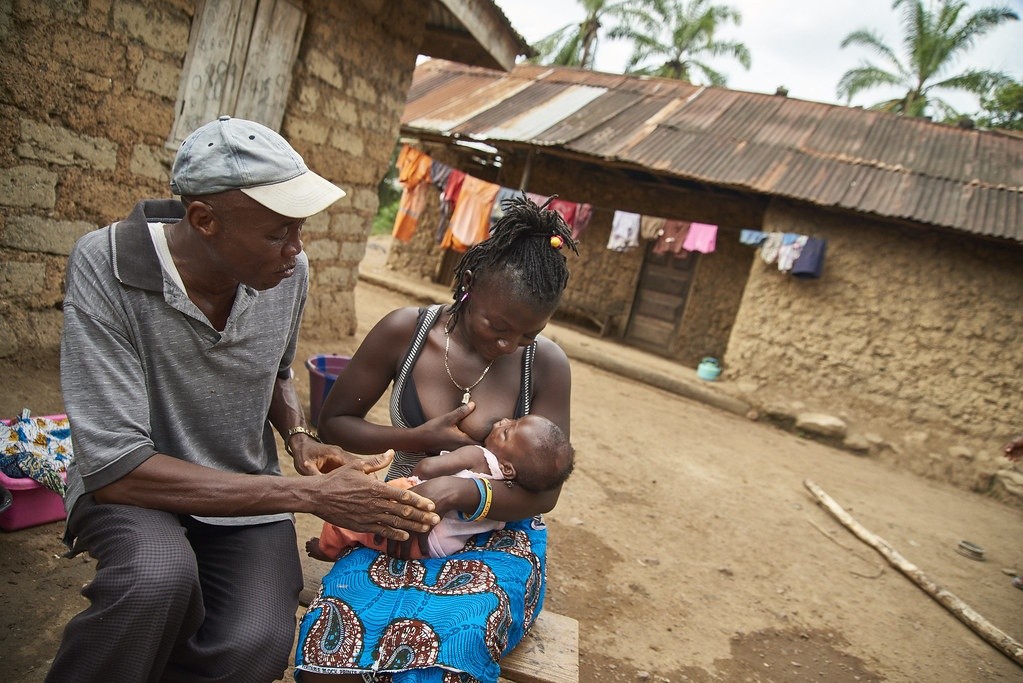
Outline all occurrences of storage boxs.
[0,412,67,532]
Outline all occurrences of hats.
[169,116,347,218]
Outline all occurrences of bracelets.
[458,478,486,521]
[285,428,322,457]
[464,478,492,522]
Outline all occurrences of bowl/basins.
[697,362,722,381]
[957,540,986,557]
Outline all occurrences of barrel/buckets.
[305,355,352,426]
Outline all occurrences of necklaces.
[444,313,495,403]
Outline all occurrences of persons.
[46,115,440,683]
[292,197,578,683]
[305,415,575,562]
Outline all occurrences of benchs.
[298,541,579,683]
